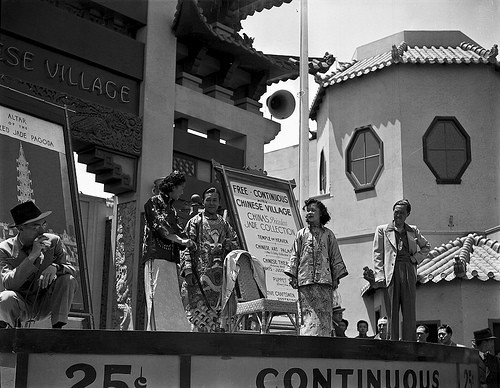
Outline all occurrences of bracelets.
[40,275,43,280]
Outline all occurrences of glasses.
[33,223,47,230]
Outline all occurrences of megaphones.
[266,90,297,119]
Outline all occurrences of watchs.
[52,263,59,271]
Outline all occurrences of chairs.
[222,250,300,336]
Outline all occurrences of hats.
[333,305,345,312]
[7,200,53,229]
[471,327,496,342]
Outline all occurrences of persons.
[0,202,77,328]
[373,200,430,340]
[332,305,348,337]
[416,326,429,343]
[437,325,455,345]
[143,172,192,332]
[373,318,387,339]
[355,320,372,338]
[473,328,500,388]
[283,198,348,337]
[182,187,240,333]
